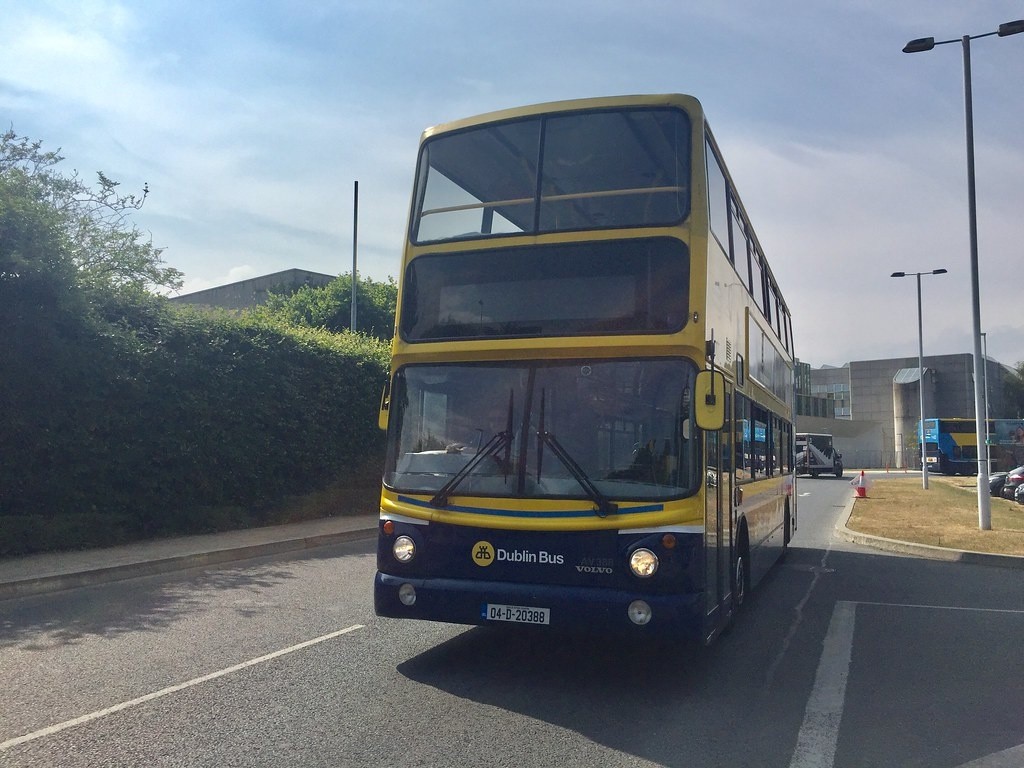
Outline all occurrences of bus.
[373,94,799,659]
[918,418,1024,477]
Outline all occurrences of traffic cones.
[853,470,870,498]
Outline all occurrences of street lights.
[900,18,1023,533]
[890,269,949,491]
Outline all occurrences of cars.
[988,465,1024,505]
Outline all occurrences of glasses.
[487,417,507,424]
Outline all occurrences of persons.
[445,409,518,461]
[1009,427,1024,445]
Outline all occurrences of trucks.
[796,432,844,479]
